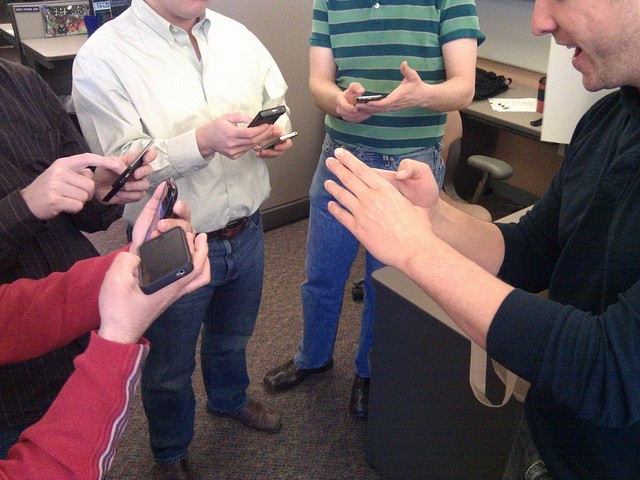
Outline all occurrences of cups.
[536,77,547,112]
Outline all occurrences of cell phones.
[143,177,178,242]
[247,105,286,125]
[136,226,195,296]
[102,140,160,202]
[256,131,299,154]
[357,95,383,103]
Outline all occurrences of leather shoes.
[350,377,369,418]
[264,357,333,389]
[206,397,282,434]
[155,456,194,480]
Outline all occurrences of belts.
[196,217,249,241]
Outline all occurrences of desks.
[0,0,89,96]
[0,22,16,42]
[453,56,569,200]
[366,205,549,478]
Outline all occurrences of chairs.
[352,139,513,301]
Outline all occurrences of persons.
[265,1,485,422]
[0,178,210,479]
[72,0,292,480]
[325,0,640,478]
[0,57,156,451]
